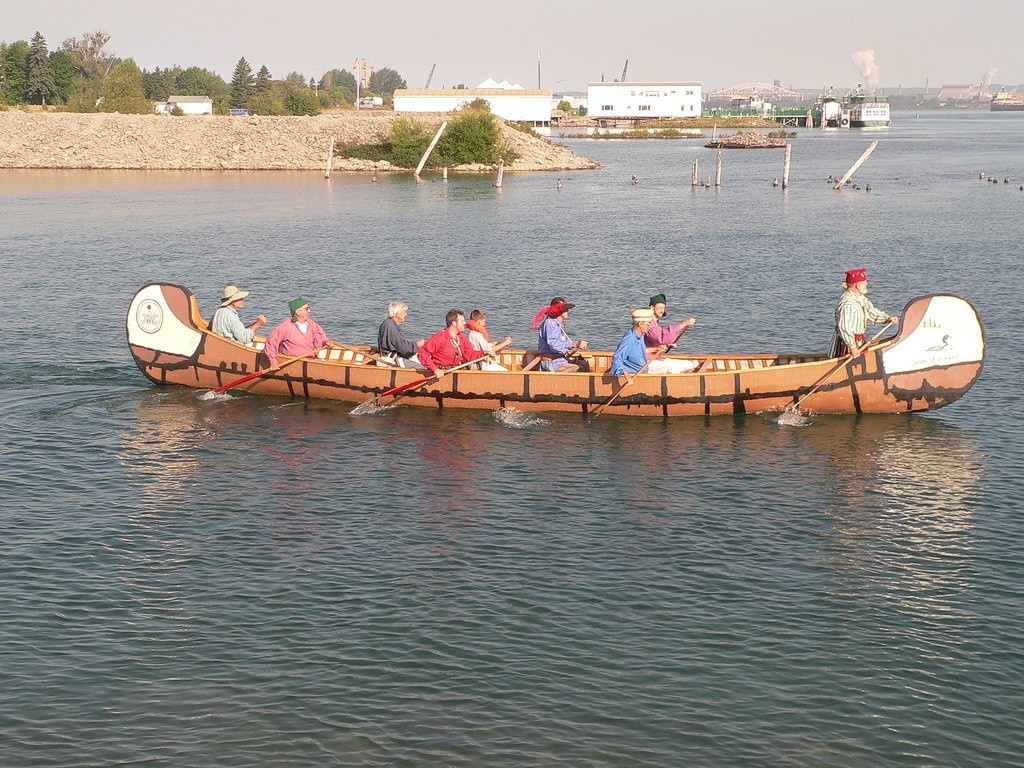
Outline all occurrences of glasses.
[302,306,310,310]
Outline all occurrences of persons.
[611,306,666,386]
[376,301,428,369]
[417,308,497,379]
[538,297,590,372]
[265,297,334,371]
[462,310,512,371]
[212,286,267,345]
[827,268,898,359]
[643,294,696,374]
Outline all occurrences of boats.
[809,94,841,127]
[989,89,1024,111]
[840,95,892,127]
[127,280,988,416]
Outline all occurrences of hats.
[844,269,867,283]
[629,307,654,322]
[219,286,249,306]
[545,297,575,317]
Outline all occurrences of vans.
[391,99,394,108]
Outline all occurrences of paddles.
[791,322,893,409]
[381,353,491,397]
[245,321,258,329]
[588,349,663,424]
[214,344,328,392]
[664,324,692,354]
[328,338,400,368]
[567,343,588,358]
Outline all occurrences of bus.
[226,108,250,116]
